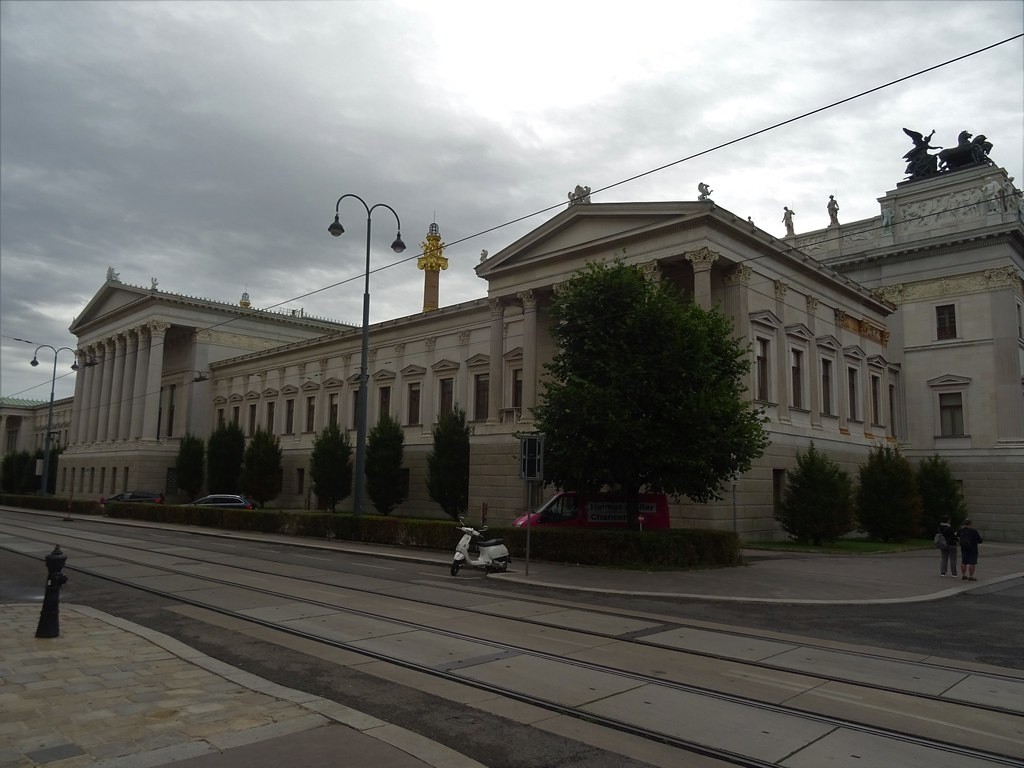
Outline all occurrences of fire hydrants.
[33,541,68,639]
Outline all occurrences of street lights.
[29,344,79,509]
[326,192,408,539]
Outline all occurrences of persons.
[747,216,754,225]
[826,195,840,227]
[782,207,795,236]
[937,515,960,577]
[956,518,982,581]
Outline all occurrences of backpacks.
[959,536,978,556]
[933,525,949,551]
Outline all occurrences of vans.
[513,489,672,534]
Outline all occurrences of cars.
[180,493,259,511]
[103,490,164,504]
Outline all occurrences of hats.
[942,515,952,521]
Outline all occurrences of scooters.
[449,516,512,577]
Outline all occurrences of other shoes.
[969,577,977,581]
[940,572,946,576]
[953,574,957,578]
[962,575,968,580]
